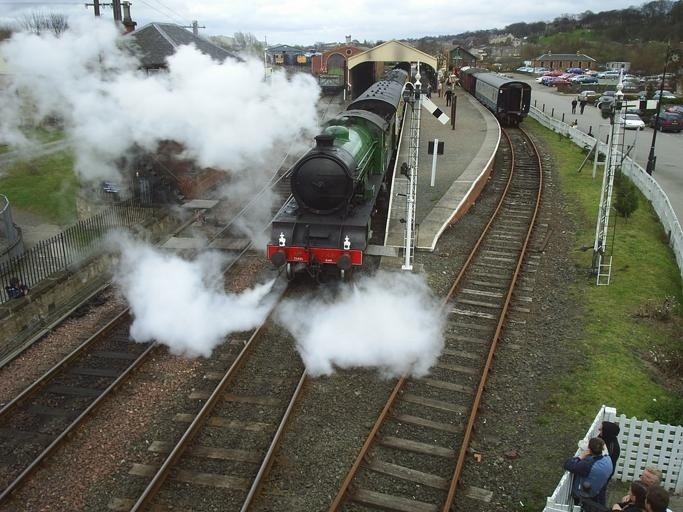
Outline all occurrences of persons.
[578,96,586,115]
[5,278,30,299]
[425,80,457,106]
[563,421,673,512]
[571,97,577,114]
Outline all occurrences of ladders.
[597,99,628,287]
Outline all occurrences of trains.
[458,63,531,126]
[266,62,412,281]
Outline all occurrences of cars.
[517,66,683,132]
